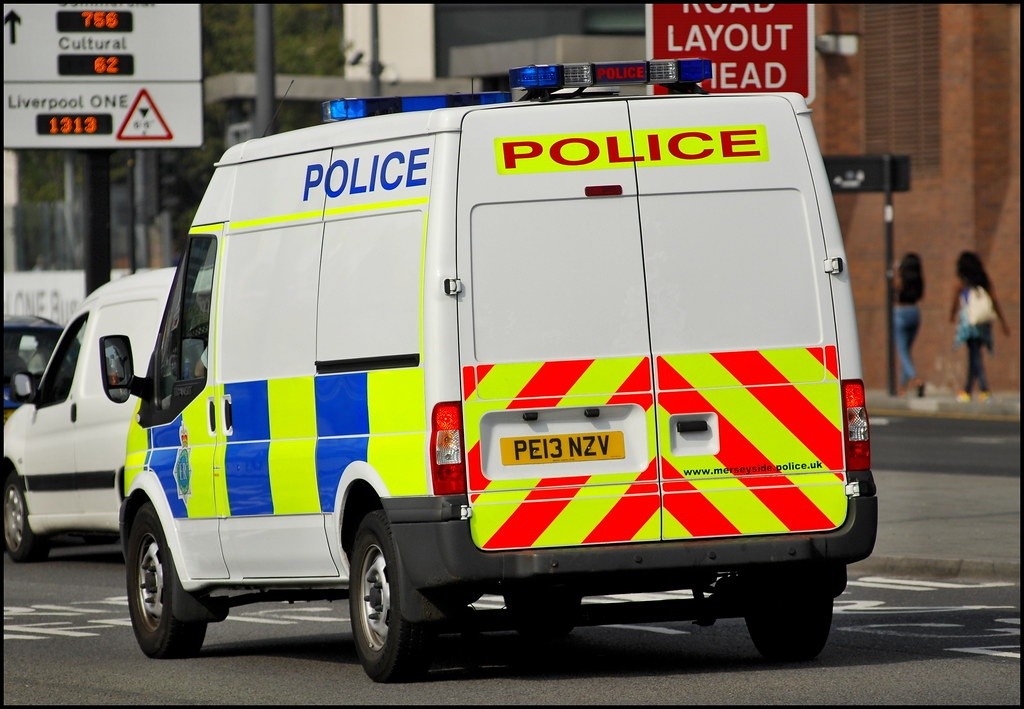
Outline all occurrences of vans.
[99,57,879,683]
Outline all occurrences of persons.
[195,348,208,376]
[950,249,1009,403]
[893,253,925,398]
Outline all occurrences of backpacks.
[959,287,997,326]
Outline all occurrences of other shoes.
[955,389,971,402]
[916,377,924,396]
[897,386,905,396]
[978,392,993,403]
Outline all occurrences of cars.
[3,314,81,425]
[3,266,176,562]
